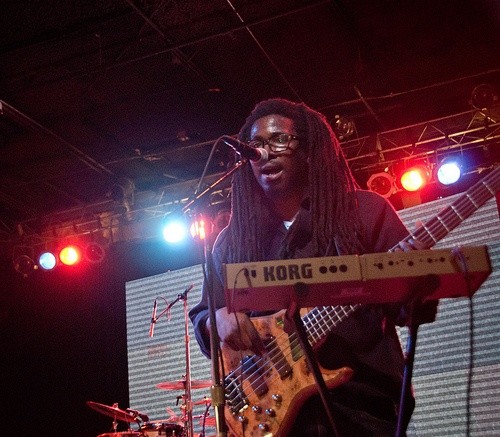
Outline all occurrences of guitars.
[215,168,500,437]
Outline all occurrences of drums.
[96,420,188,437]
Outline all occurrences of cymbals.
[87,400,142,424]
[156,380,212,390]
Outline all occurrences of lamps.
[13,228,106,278]
[366,151,469,198]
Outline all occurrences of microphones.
[127,409,149,422]
[149,300,157,338]
[223,135,268,166]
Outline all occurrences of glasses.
[248,133,310,153]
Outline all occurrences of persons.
[189,98,440,437]
